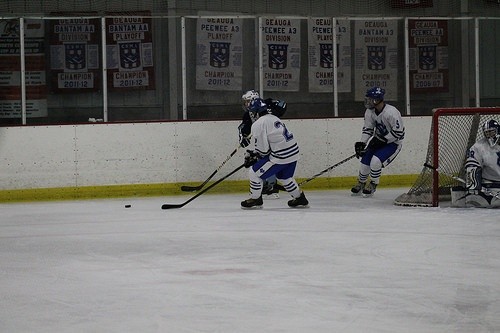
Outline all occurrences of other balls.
[125,205,131,208]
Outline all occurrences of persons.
[451,119,500,209]
[240,98,310,208]
[351,86,406,198]
[238,90,287,200]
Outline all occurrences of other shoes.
[249,179,279,199]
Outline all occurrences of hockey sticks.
[423,163,500,199]
[272,154,357,192]
[181,146,241,192]
[161,163,245,209]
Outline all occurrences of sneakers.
[361,181,378,197]
[240,193,264,210]
[350,180,366,196]
[287,191,310,209]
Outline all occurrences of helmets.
[249,98,267,120]
[241,90,259,110]
[364,87,385,110]
[482,120,500,148]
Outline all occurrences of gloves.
[367,134,387,150]
[239,134,251,148]
[244,150,258,168]
[355,142,366,159]
